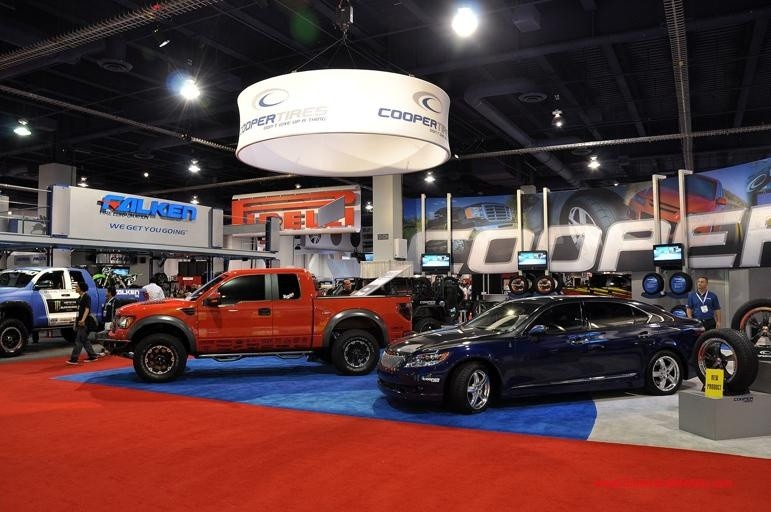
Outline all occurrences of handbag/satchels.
[85,314,98,332]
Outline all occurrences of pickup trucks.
[98,267,415,382]
[1,266,148,358]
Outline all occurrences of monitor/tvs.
[364,253,374,260]
[653,243,683,263]
[517,250,547,269]
[110,267,129,276]
[420,253,450,269]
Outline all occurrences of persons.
[685,276,721,330]
[95,285,118,358]
[65,281,99,365]
[141,278,197,302]
[326,278,358,297]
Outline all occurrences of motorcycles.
[93,265,146,289]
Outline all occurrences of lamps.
[230,2,452,180]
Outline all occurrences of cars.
[626,174,728,239]
[376,294,707,414]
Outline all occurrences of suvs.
[426,203,517,262]
[318,278,356,297]
[328,273,462,334]
